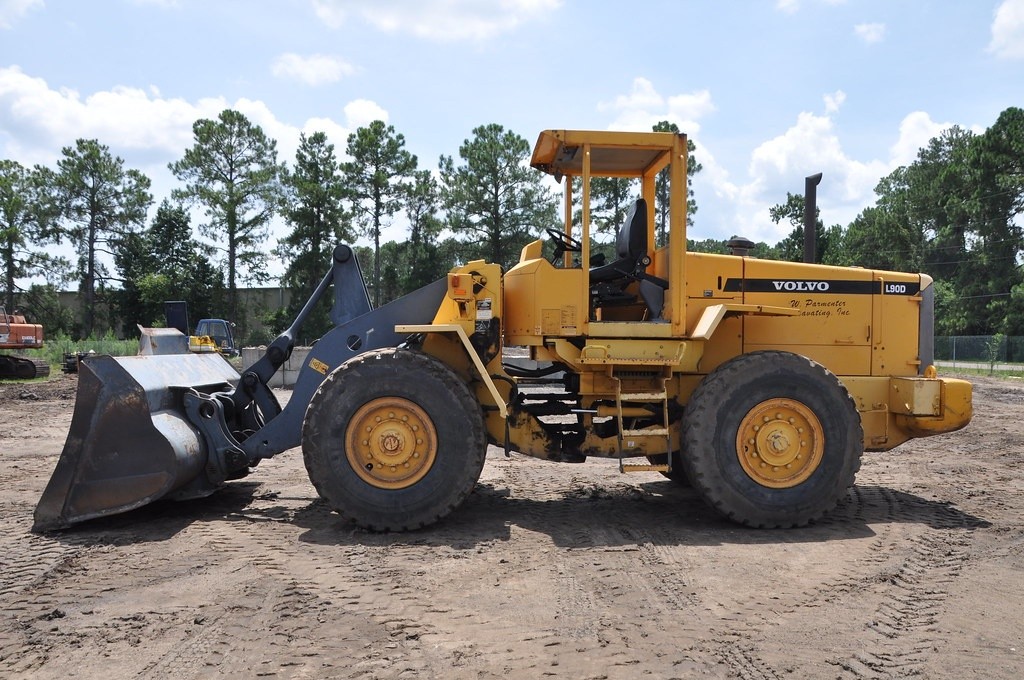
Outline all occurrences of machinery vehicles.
[25,129,979,528]
[0,306,50,379]
[185,318,235,358]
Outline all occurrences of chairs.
[588,198,649,290]
